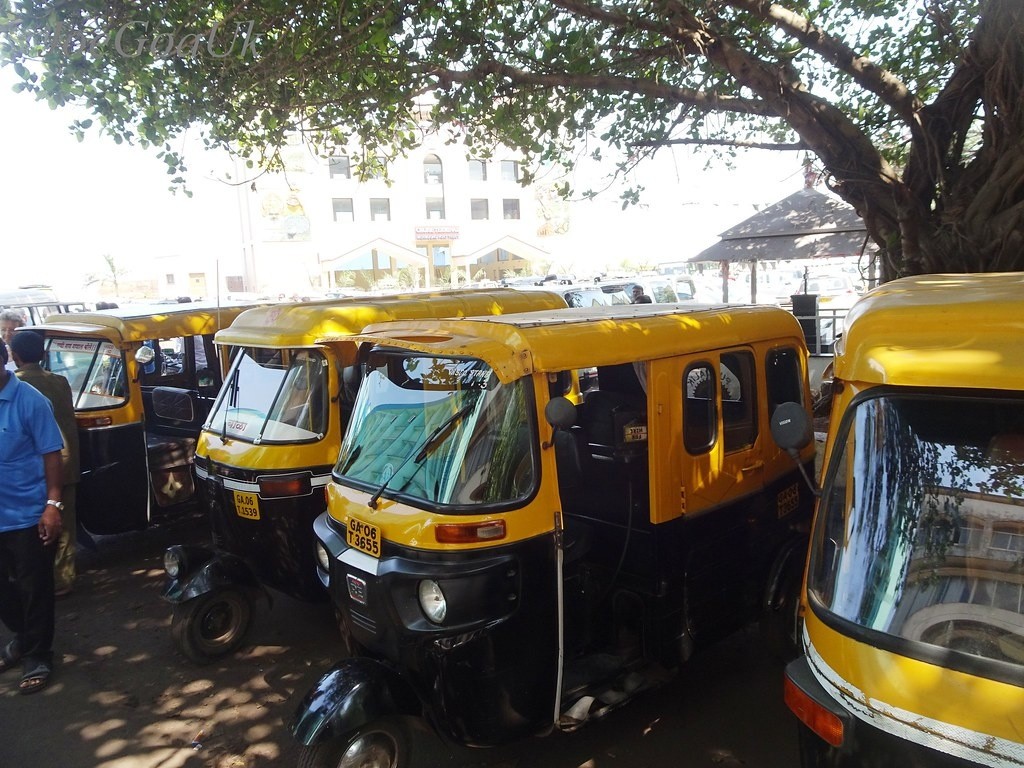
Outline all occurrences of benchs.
[139,384,200,472]
[579,446,652,578]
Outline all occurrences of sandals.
[19,659,52,696]
[0,638,22,674]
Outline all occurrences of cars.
[520,275,714,310]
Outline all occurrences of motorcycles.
[284,303,817,768]
[0,289,570,768]
[771,270,1024,768]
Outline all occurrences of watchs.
[46,499,65,511]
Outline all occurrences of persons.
[0,338,65,693]
[9,331,89,595]
[593,276,600,285]
[95,301,120,371]
[631,284,652,303]
[177,297,207,374]
[534,279,573,286]
[0,310,27,372]
[264,293,311,302]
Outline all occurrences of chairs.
[581,389,639,446]
